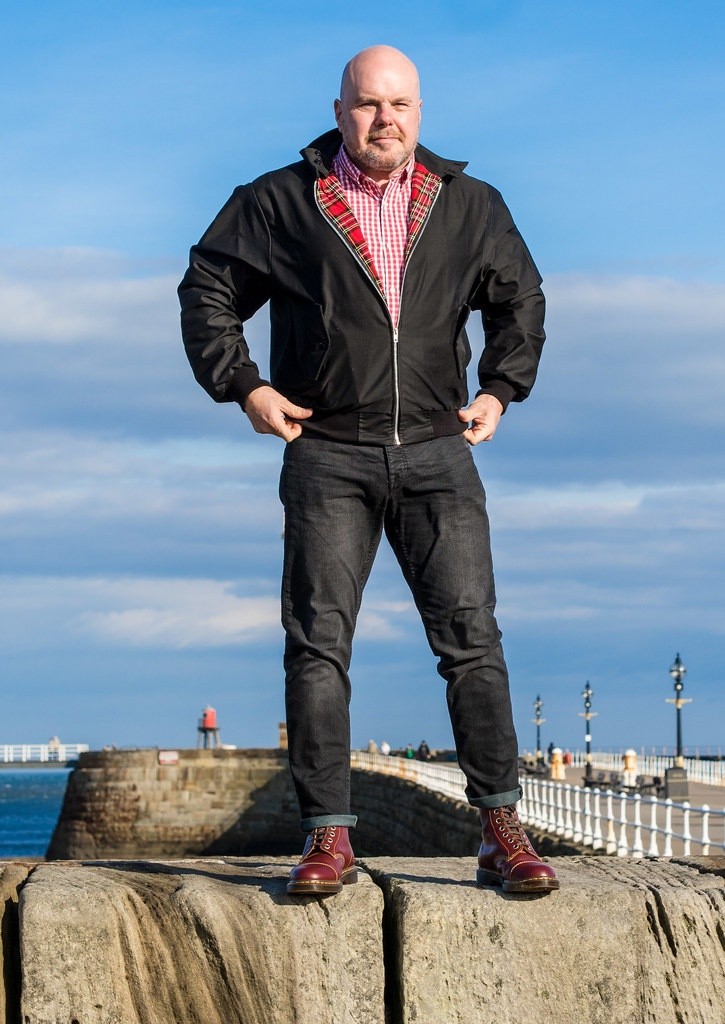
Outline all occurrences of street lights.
[666,653,693,770]
[578,681,598,790]
[532,694,546,774]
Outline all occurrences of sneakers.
[476,803,560,893]
[285,826,358,892]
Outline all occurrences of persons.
[177,46,559,899]
